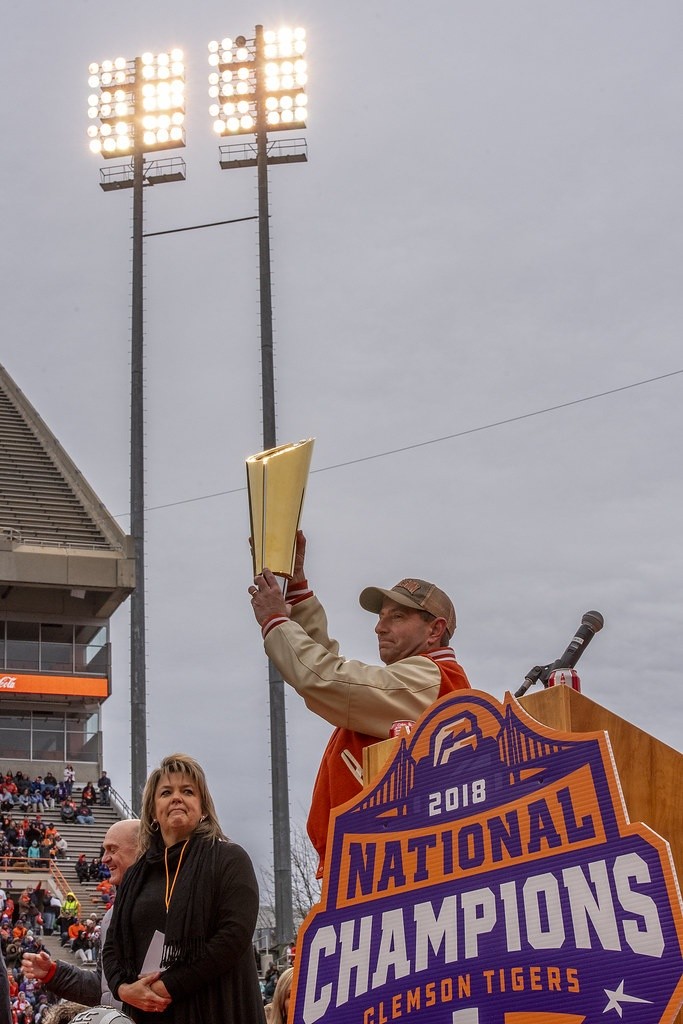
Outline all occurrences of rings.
[21,967,25,973]
[154,1007,158,1012]
[251,589,257,594]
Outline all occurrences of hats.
[0,765,293,1024]
[359,578,455,637]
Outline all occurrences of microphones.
[548,610,604,680]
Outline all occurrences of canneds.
[388,720,416,740]
[547,668,581,695]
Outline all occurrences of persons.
[0,763,116,1024]
[102,754,267,1024]
[20,819,149,1014]
[248,530,471,882]
[264,942,297,1024]
